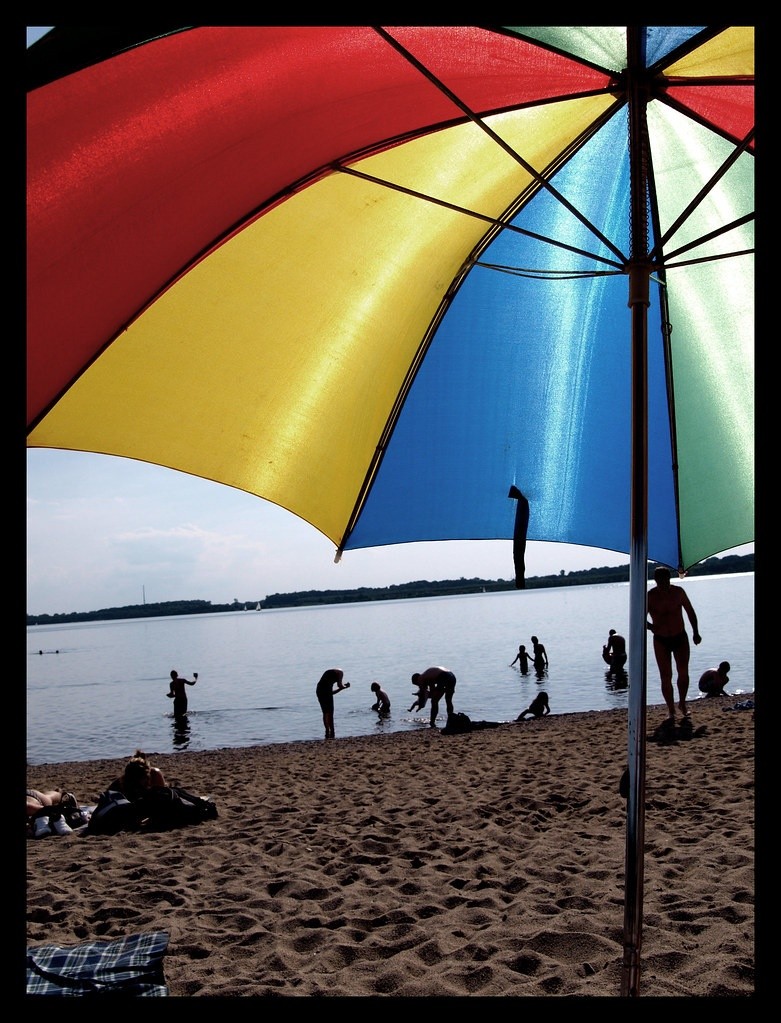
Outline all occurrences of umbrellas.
[28,25,753,995]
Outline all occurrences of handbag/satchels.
[85,787,219,834]
[441,712,472,735]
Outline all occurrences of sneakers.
[34,816,51,836]
[54,814,73,835]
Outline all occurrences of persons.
[316,669,350,738]
[531,635,548,665]
[407,666,456,727]
[166,669,199,716]
[647,566,701,718]
[698,662,731,697]
[510,645,534,674]
[515,691,550,721]
[602,629,627,673]
[89,752,165,831]
[27,788,78,835]
[370,683,391,712]
